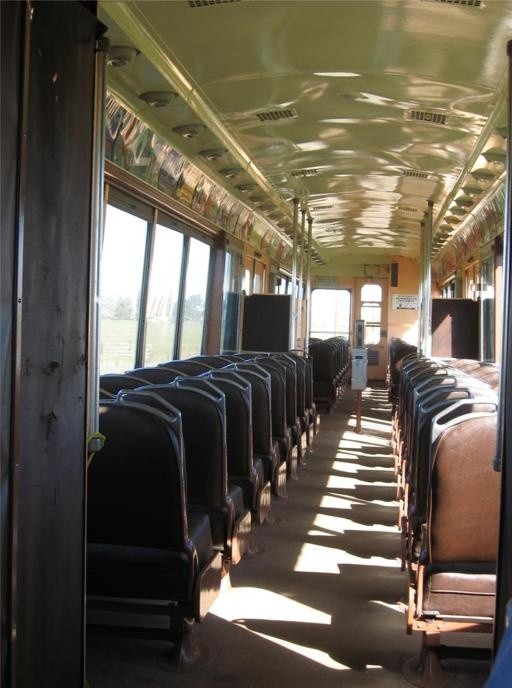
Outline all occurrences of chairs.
[384,338,509,688]
[93,334,350,675]
